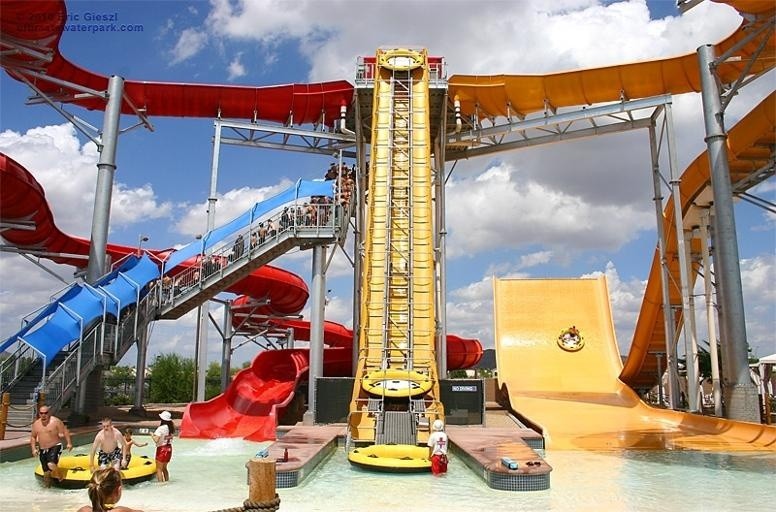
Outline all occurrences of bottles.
[283,448,288,462]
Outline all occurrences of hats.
[158,411,172,421]
[434,420,444,431]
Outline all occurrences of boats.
[33,452,157,491]
[379,48,423,72]
[361,370,434,402]
[557,329,585,352]
[348,441,433,472]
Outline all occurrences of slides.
[180,350,300,443]
[352,48,446,469]
[493,278,775,457]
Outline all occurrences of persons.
[150,410,172,484]
[73,467,146,512]
[119,159,357,319]
[122,428,148,469]
[427,420,448,476]
[30,405,72,487]
[89,416,126,472]
[561,325,581,348]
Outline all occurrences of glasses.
[39,411,48,414]
